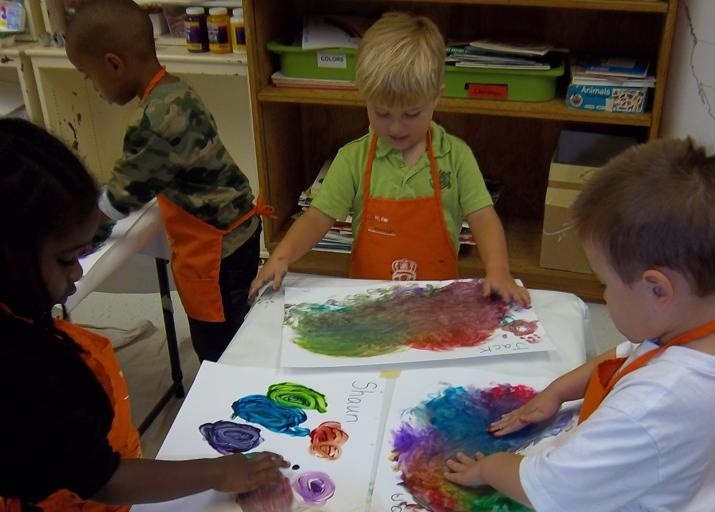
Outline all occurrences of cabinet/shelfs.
[0,1,50,140]
[27,0,266,260]
[243,0,683,305]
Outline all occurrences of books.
[443,32,657,90]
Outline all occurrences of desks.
[131,271,594,512]
[56,189,186,447]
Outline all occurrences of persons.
[246,13,533,306]
[441,137,715,511]
[1,115,292,510]
[61,0,265,359]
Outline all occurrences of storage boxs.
[543,128,641,271]
[268,36,360,82]
[567,78,651,114]
[437,53,567,104]
